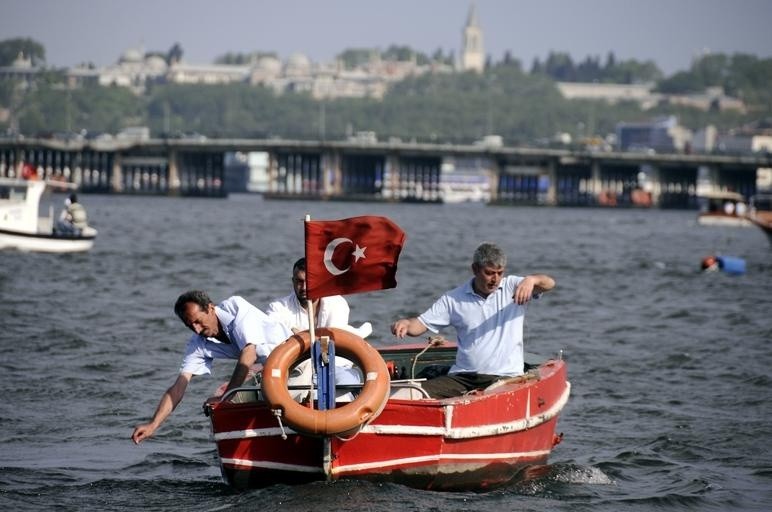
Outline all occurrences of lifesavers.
[261,328,390,434]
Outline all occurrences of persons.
[58,193,86,237]
[264,257,365,403]
[60,197,73,222]
[132,290,313,446]
[391,242,556,398]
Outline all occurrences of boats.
[0,176,96,257]
[202,335,572,494]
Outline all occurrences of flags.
[304,215,406,300]
